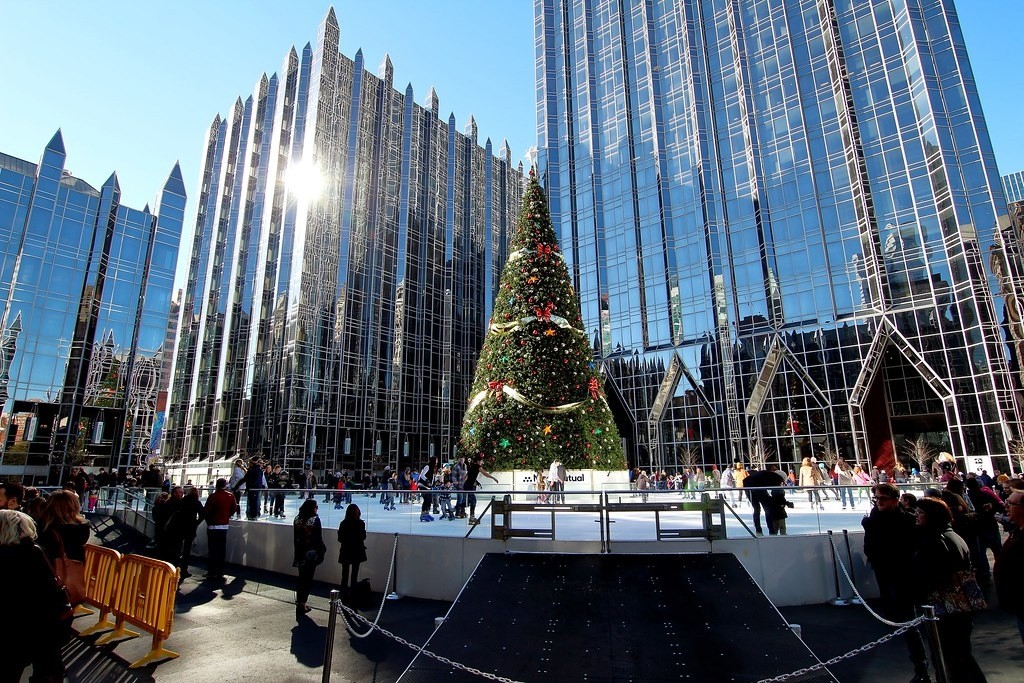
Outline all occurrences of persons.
[0,452,1024,683]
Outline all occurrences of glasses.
[872,496,895,503]
[1004,498,1024,507]
[913,509,924,515]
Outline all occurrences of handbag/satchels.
[50,526,86,607]
[914,532,989,619]
[307,550,324,565]
[35,544,72,603]
[352,545,367,561]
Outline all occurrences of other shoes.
[305,606,311,613]
[469,518,477,525]
[206,572,223,578]
[181,572,192,578]
[107,501,113,505]
[909,671,932,683]
[296,615,312,625]
[129,503,132,506]
